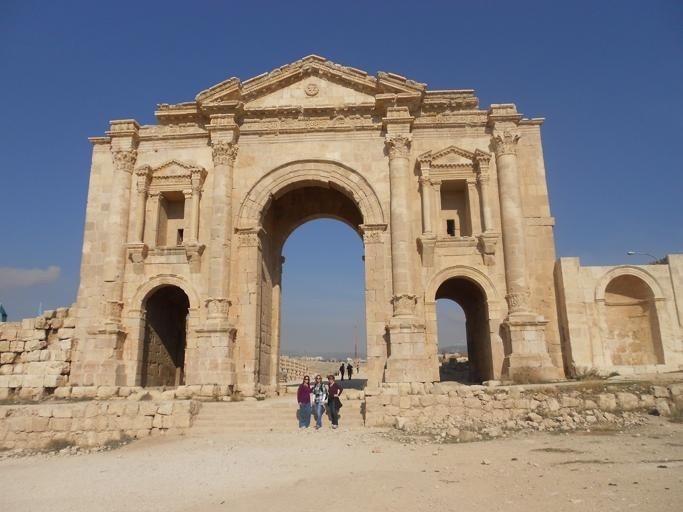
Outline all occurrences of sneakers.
[332,425,338,429]
[299,425,322,430]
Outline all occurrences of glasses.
[304,379,309,380]
[317,378,321,379]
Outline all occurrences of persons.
[325,375,343,429]
[338,363,345,381]
[355,361,359,373]
[296,375,313,429]
[310,374,327,429]
[346,363,353,380]
